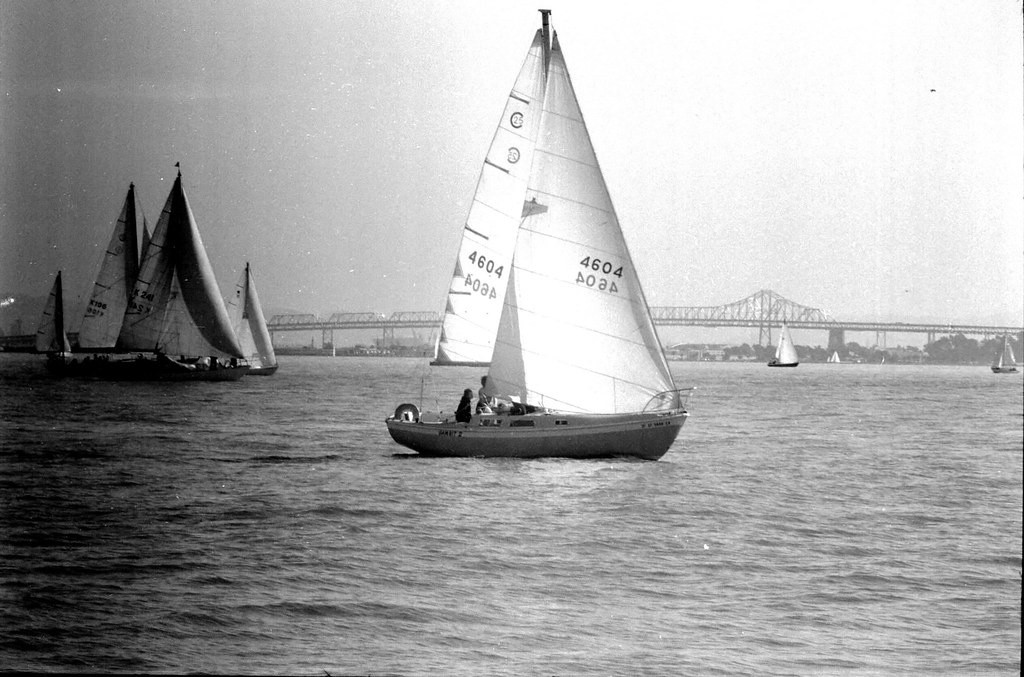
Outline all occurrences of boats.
[991,367,1020,373]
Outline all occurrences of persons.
[456,389,473,423]
[231,358,237,366]
[475,376,498,425]
[210,356,218,370]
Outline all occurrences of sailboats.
[768,319,799,367]
[386,8,696,462]
[825,351,841,364]
[28,162,278,384]
